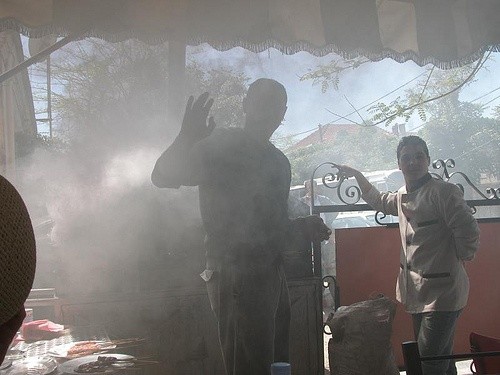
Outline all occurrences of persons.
[299,180,339,277]
[151,78,332,375]
[330,136,481,375]
[0,176,36,368]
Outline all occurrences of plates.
[47,342,116,357]
[5,356,57,375]
[58,353,137,374]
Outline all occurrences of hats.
[0,175,37,325]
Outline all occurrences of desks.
[0,325,128,375]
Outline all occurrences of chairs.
[470,331,500,375]
